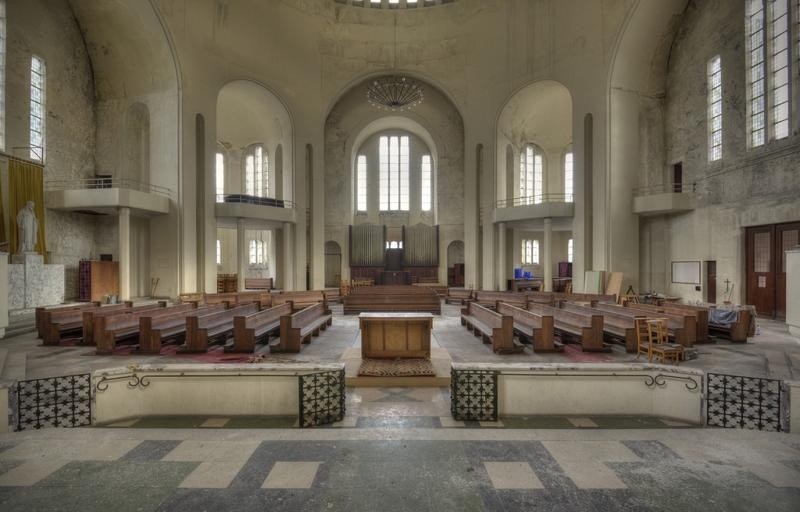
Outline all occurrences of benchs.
[449,279,752,352]
[345,283,442,317]
[38,278,343,356]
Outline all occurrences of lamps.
[366,13,424,113]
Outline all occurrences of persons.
[16,201,39,252]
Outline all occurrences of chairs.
[636,318,681,366]
